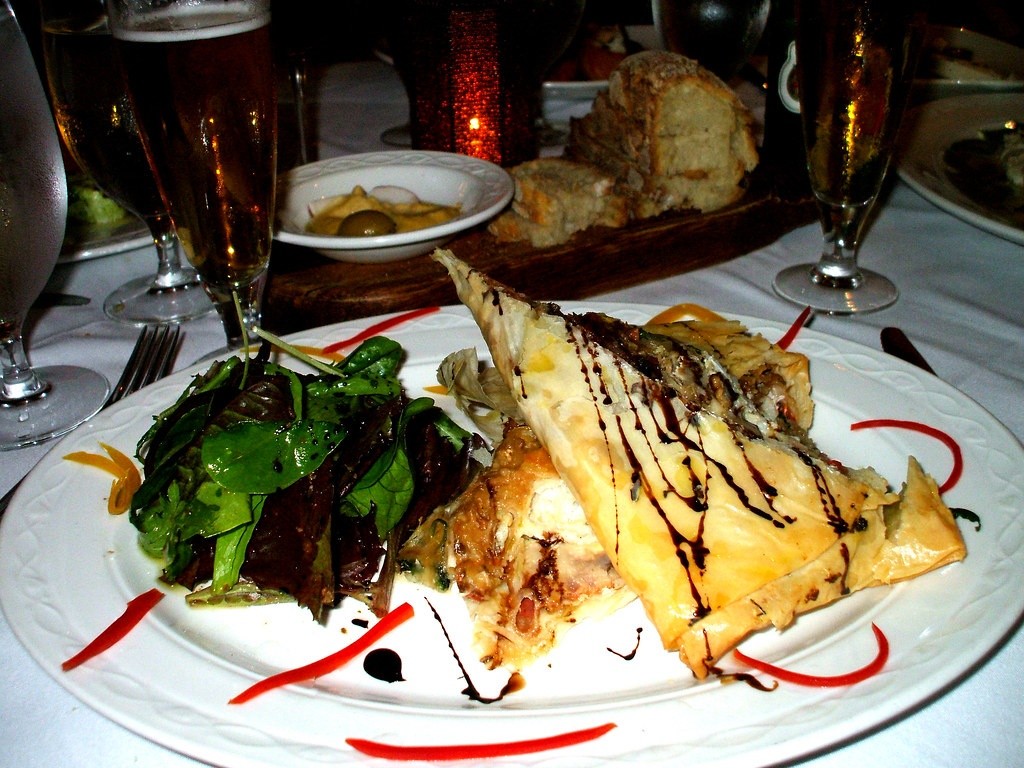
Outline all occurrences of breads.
[488,48,761,254]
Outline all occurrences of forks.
[1,323,182,520]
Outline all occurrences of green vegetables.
[132,291,493,624]
[65,167,135,224]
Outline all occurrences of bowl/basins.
[536,78,610,135]
[273,148,514,265]
[922,24,1024,99]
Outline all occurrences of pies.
[396,248,966,675]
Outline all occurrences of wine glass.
[769,0,917,317]
[105,0,282,371]
[11,0,218,328]
[0,0,112,455]
[379,0,441,147]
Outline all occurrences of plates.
[896,90,1024,248]
[0,300,1024,768]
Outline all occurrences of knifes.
[879,324,935,378]
